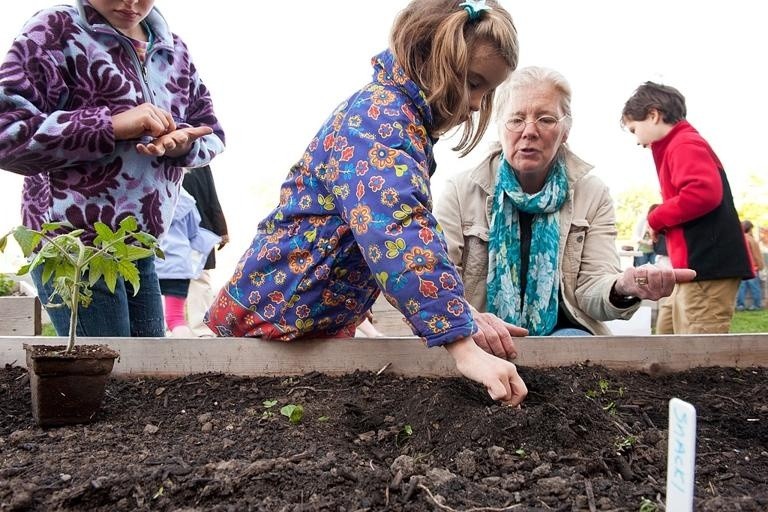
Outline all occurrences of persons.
[620,82,755,334]
[610,201,767,324]
[197,2,530,407]
[432,65,698,360]
[1,0,225,336]
[180,166,230,334]
[151,160,224,333]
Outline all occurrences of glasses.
[502,115,566,132]
